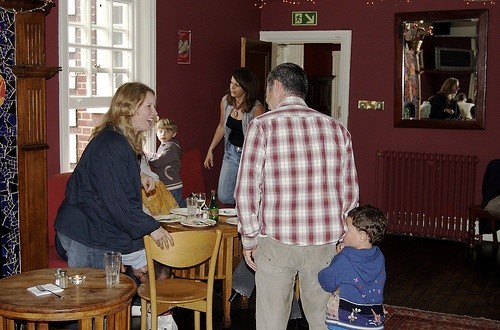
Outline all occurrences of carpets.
[382,304,500,330]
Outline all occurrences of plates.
[226,217,238,225]
[218,208,237,216]
[154,214,186,223]
[181,219,216,227]
[170,208,203,216]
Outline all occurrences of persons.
[54,82,179,330]
[147,119,183,203]
[204,67,265,204]
[428,77,475,120]
[232,63,359,330]
[318,204,388,330]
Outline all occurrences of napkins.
[27,283,64,296]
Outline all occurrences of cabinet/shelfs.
[8,12,63,272]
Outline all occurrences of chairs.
[141,182,179,216]
[137,230,233,330]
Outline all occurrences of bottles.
[209,190,219,221]
[55,268,68,288]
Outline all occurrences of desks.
[0,267,137,330]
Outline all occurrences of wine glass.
[194,192,206,218]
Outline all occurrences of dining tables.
[160,213,248,310]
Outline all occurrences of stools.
[467,205,500,251]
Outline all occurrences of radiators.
[376,151,478,240]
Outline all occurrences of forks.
[35,284,64,299]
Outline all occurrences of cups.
[187,197,197,219]
[106,251,121,285]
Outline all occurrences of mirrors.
[394,8,488,130]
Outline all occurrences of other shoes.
[213,285,240,303]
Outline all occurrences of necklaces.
[234,111,238,117]
